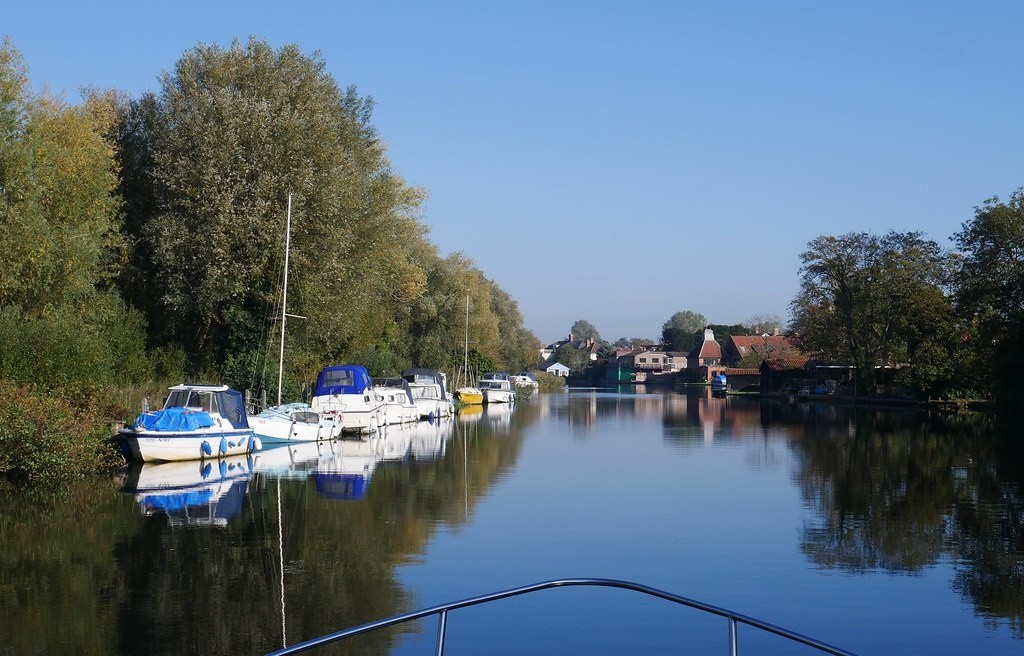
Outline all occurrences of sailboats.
[246,191,348,448]
[453,294,485,405]
[459,404,482,521]
[250,437,337,648]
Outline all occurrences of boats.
[117,383,263,464]
[478,371,539,403]
[135,453,254,527]
[711,384,728,393]
[712,373,727,384]
[309,366,459,436]
